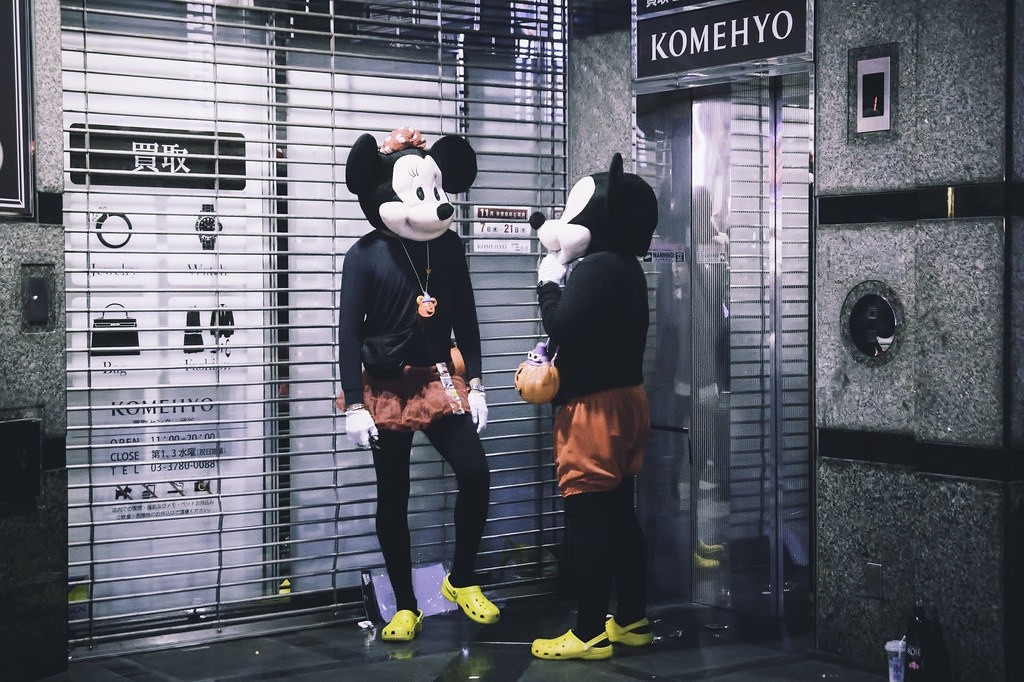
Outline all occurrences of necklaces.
[397,235,437,317]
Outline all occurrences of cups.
[884,639,906,682]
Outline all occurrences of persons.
[335,131,503,641]
[510,151,656,663]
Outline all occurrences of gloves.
[468,392,488,435]
[537,251,567,285]
[345,407,380,450]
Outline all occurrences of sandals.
[381,609,424,641]
[694,555,720,569]
[605,616,653,646]
[441,573,500,625]
[531,629,613,660]
[696,540,724,556]
